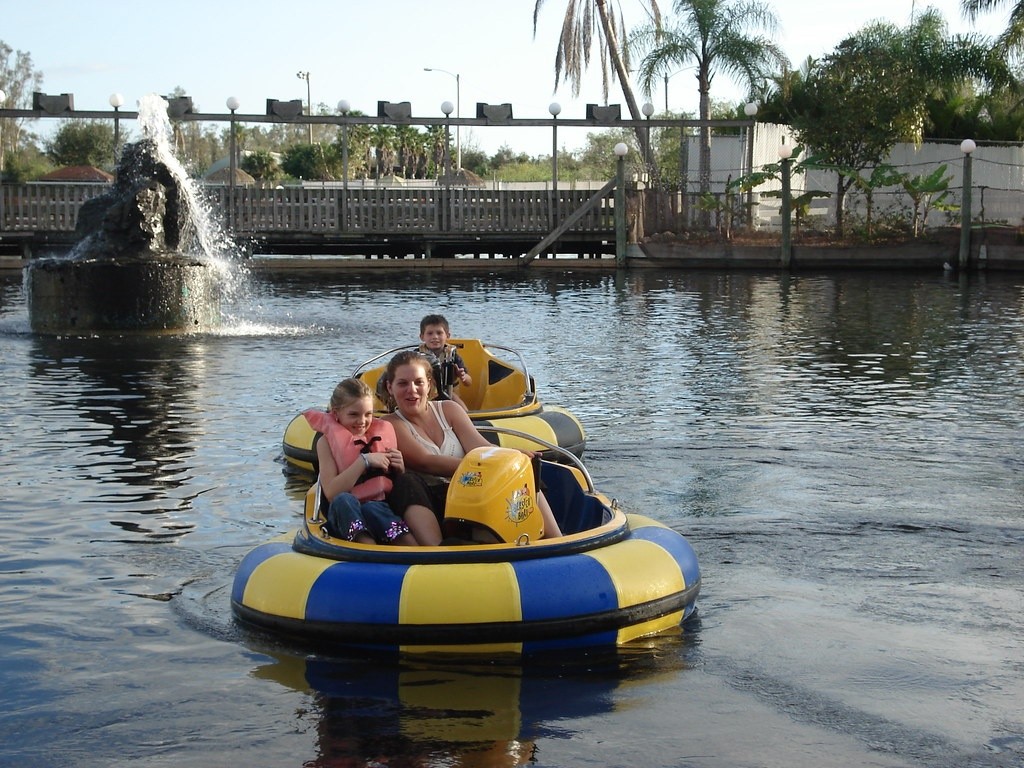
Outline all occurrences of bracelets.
[361,453,370,467]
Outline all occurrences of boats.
[228,425,703,657]
[282,341,586,472]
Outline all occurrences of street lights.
[614,143,628,270]
[337,99,351,187]
[424,68,461,169]
[627,66,701,130]
[226,96,241,186]
[297,71,313,146]
[109,93,124,183]
[743,101,760,231]
[777,144,793,261]
[441,101,455,186]
[958,138,977,262]
[548,103,561,202]
[642,103,655,165]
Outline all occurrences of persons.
[380,350,562,546]
[413,314,473,414]
[316,379,420,546]
